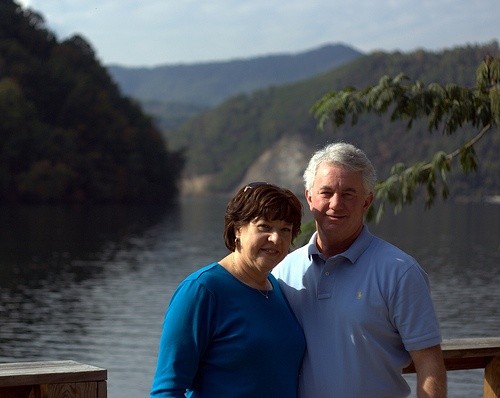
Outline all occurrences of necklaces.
[232,263,269,300]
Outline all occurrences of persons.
[269,142,448,398]
[149,182,308,398]
